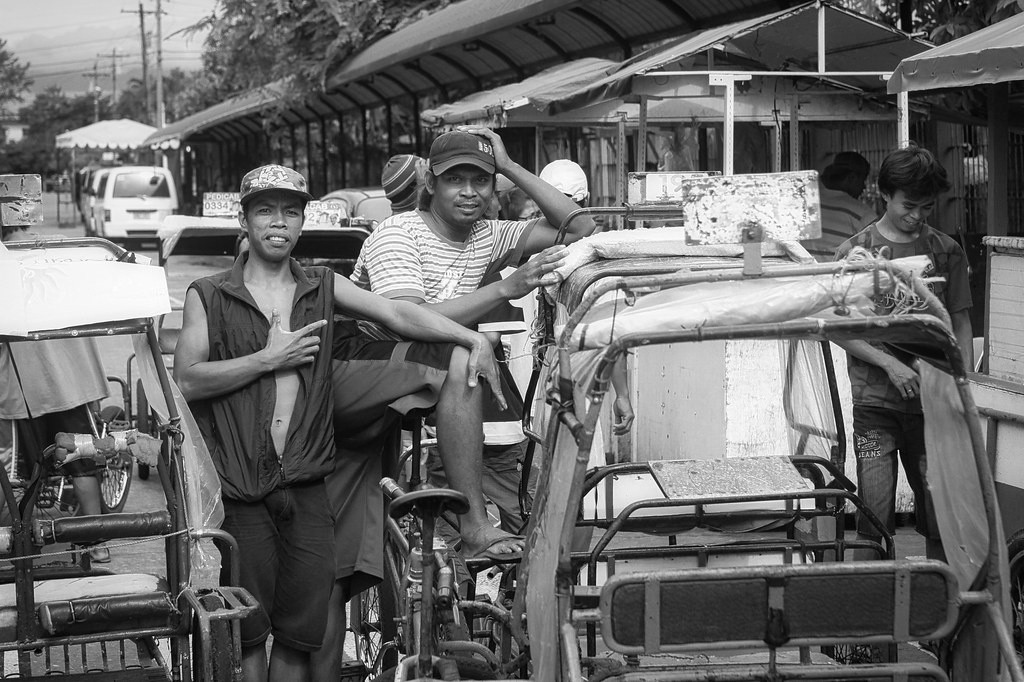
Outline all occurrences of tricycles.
[0,171,1024,681]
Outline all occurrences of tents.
[55,117,166,225]
[885,8,1024,150]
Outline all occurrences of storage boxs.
[0,173,44,227]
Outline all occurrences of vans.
[85,164,180,251]
[76,166,111,237]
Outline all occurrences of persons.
[1,225,113,563]
[175,124,635,682]
[805,144,976,652]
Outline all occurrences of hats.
[430,130,497,176]
[240,164,312,206]
[381,154,425,212]
[539,158,588,202]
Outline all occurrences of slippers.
[458,524,527,559]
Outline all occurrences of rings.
[907,388,912,393]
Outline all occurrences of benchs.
[567,454,960,682]
